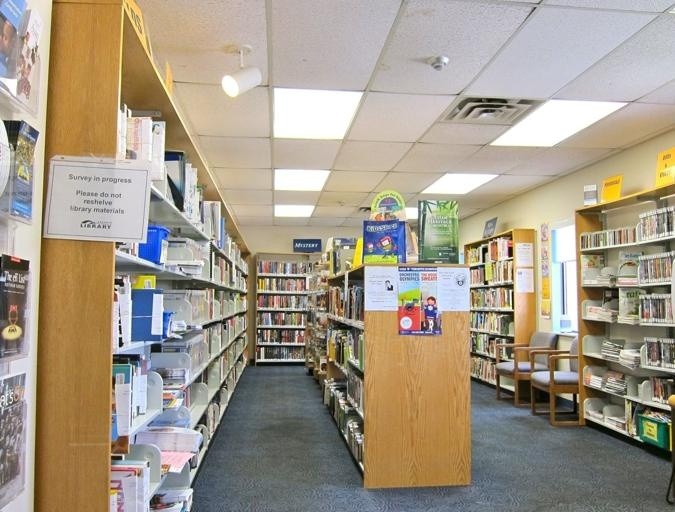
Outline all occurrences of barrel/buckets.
[131,287,164,338]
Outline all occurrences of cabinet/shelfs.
[308,261,470,490]
[35,1,252,512]
[579,181,675,453]
[256,252,311,364]
[465,229,534,397]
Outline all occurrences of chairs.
[531,336,579,426]
[495,332,558,408]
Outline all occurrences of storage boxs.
[636,411,669,450]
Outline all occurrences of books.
[415,199,459,264]
[579,205,675,453]
[321,381,364,465]
[0,0,41,512]
